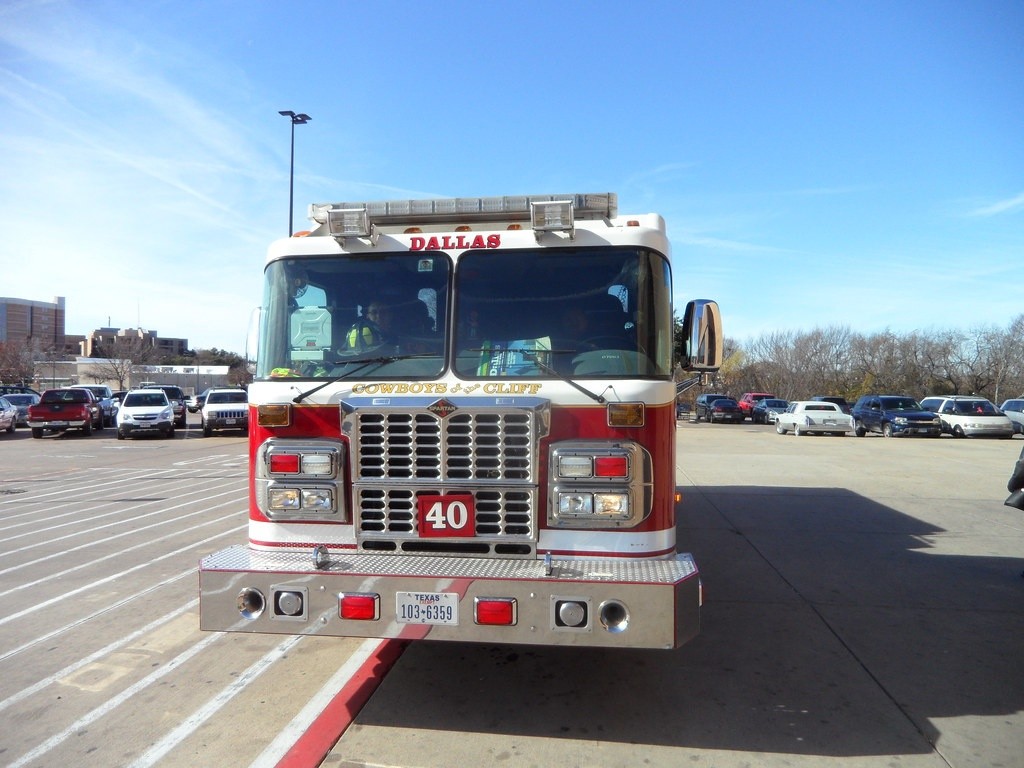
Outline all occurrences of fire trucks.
[196,193,723,650]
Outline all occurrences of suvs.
[185,386,249,414]
[808,395,851,416]
[197,386,250,436]
[113,388,177,439]
[738,392,776,421]
[138,383,195,427]
[694,393,734,420]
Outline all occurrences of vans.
[0,385,42,401]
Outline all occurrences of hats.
[582,294,630,324]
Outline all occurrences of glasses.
[366,309,388,316]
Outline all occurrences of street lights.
[279,109,312,238]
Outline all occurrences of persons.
[542,299,603,352]
[365,299,397,332]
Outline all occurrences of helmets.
[338,319,391,357]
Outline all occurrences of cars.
[751,398,794,426]
[0,396,20,435]
[918,394,1014,439]
[851,394,943,438]
[706,399,744,425]
[775,400,855,437]
[999,398,1023,435]
[58,383,129,427]
[846,401,857,414]
[2,393,40,428]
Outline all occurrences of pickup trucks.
[26,387,105,438]
